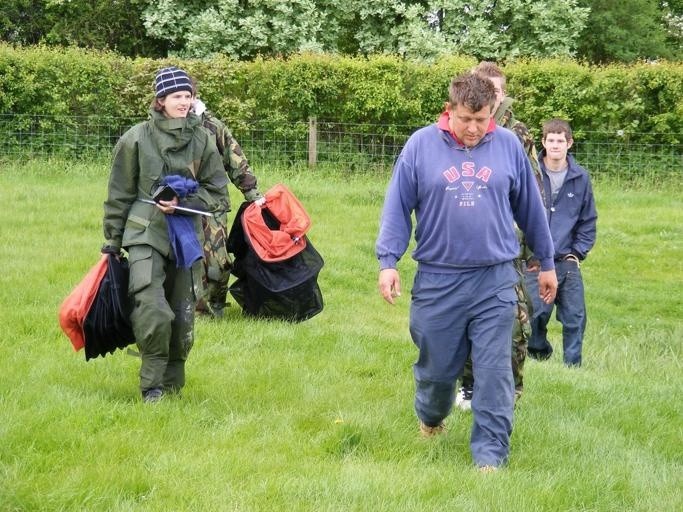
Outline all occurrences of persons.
[183,77,263,318]
[457,63,545,411]
[523,122,598,365]
[103,67,231,399]
[376,74,557,473]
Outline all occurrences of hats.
[155,66,195,99]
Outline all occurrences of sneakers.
[420,422,444,437]
[144,387,163,402]
[210,308,223,319]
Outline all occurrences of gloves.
[245,189,262,201]
